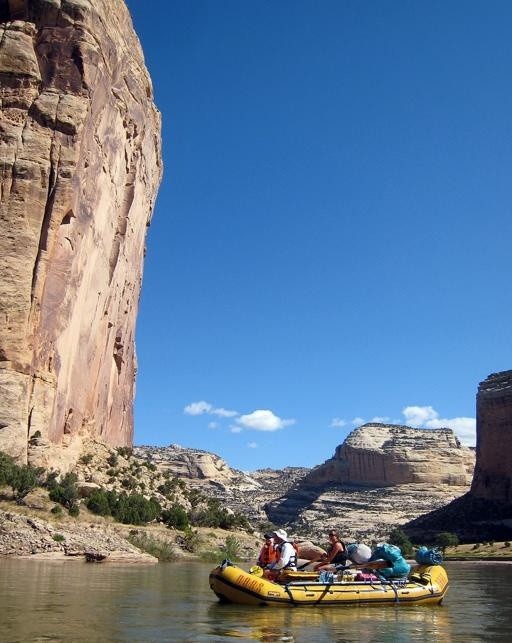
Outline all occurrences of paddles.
[327,560,395,570]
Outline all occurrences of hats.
[264,533,274,538]
[272,529,288,542]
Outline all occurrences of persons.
[264,529,297,583]
[257,533,281,577]
[313,530,348,572]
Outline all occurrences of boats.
[209,564,450,607]
